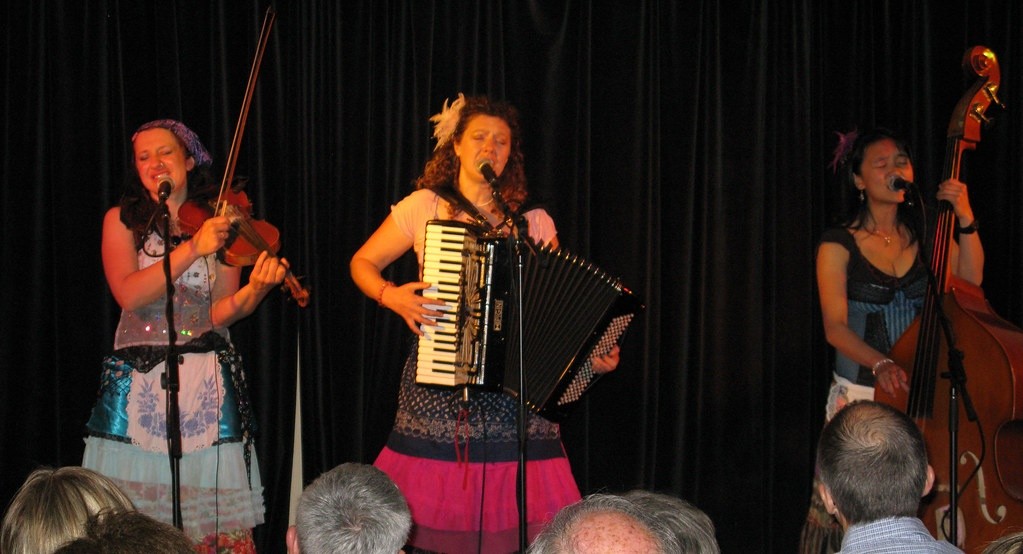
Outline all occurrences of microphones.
[886,174,918,193]
[475,158,500,189]
[156,175,176,200]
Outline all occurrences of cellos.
[869,41,1023,554]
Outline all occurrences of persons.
[83,121,290,554]
[351,92,620,554]
[799,127,982,554]
[0,400,1023,554]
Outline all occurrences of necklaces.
[477,198,494,207]
[869,221,896,244]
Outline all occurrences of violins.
[178,185,312,309]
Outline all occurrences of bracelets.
[873,359,895,376]
[378,281,396,309]
[959,219,980,234]
